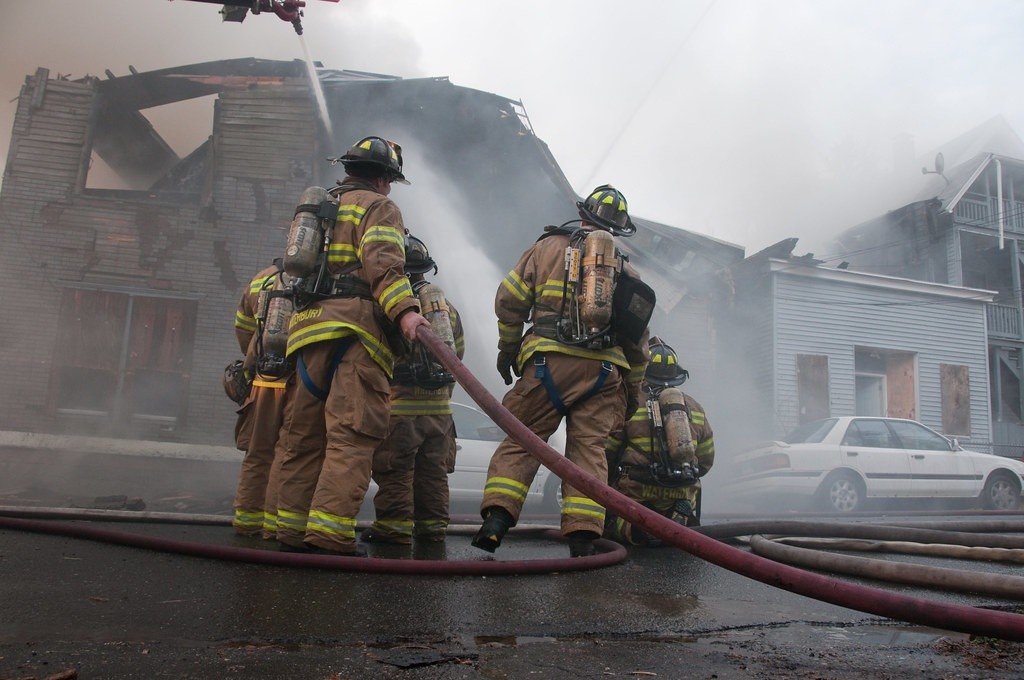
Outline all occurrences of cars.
[361,399,565,516]
[726,414,1023,520]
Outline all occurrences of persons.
[264,136,436,558]
[607,337,718,544]
[361,230,468,549]
[227,248,305,543]
[462,183,640,557]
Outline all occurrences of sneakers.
[569,533,597,557]
[637,502,663,547]
[664,500,691,527]
[362,528,410,544]
[471,508,510,554]
[307,536,368,558]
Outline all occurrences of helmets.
[404,230,436,276]
[578,184,637,238]
[328,134,410,187]
[645,335,688,387]
